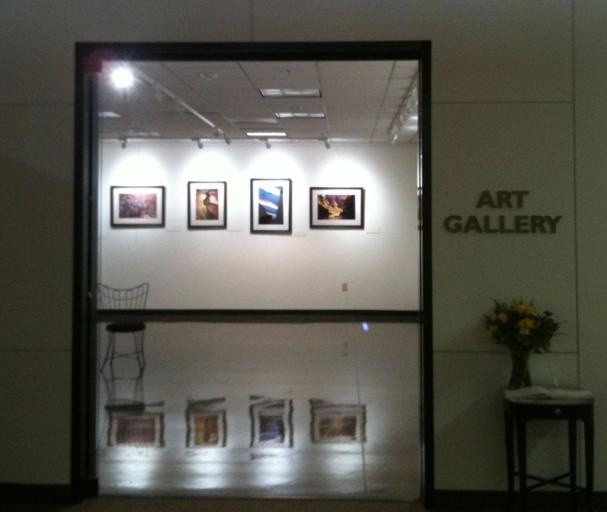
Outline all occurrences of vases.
[506,349,532,388]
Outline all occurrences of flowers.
[478,297,560,355]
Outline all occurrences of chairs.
[97,372,148,457]
[96,282,149,372]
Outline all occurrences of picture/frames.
[111,186,164,228]
[107,413,165,447]
[250,395,293,446]
[188,181,227,228]
[185,399,227,447]
[250,179,291,234]
[310,400,366,444]
[310,187,364,229]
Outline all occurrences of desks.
[504,385,594,512]
